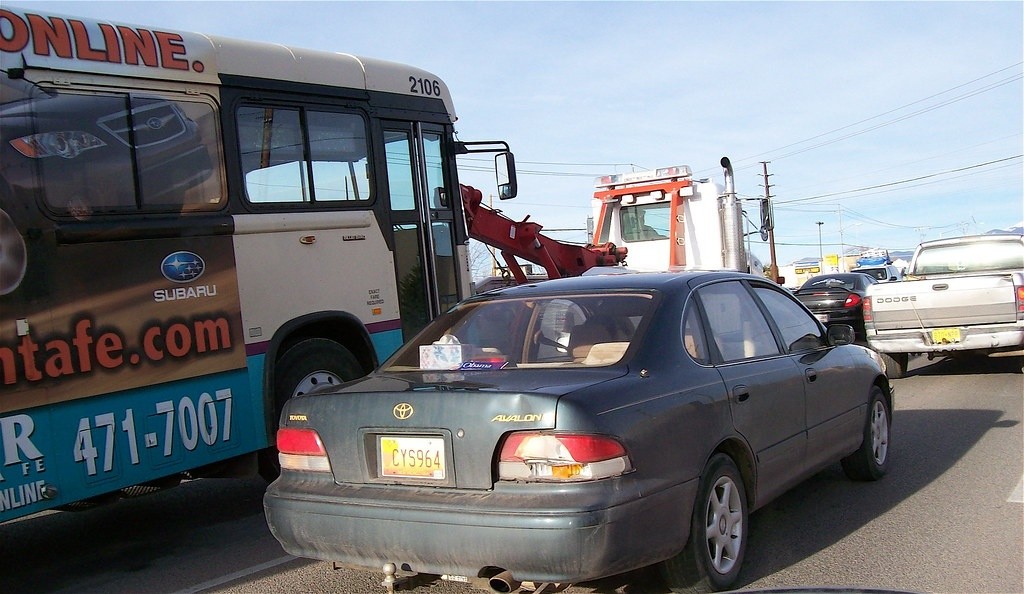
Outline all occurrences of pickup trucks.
[863,234,1024,379]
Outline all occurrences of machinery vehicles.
[433,155,785,378]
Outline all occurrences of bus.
[0,7,517,530]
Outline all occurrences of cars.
[850,265,903,287]
[788,271,885,348]
[255,273,899,594]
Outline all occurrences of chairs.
[567,313,637,364]
[685,328,726,363]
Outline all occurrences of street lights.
[815,220,826,272]
